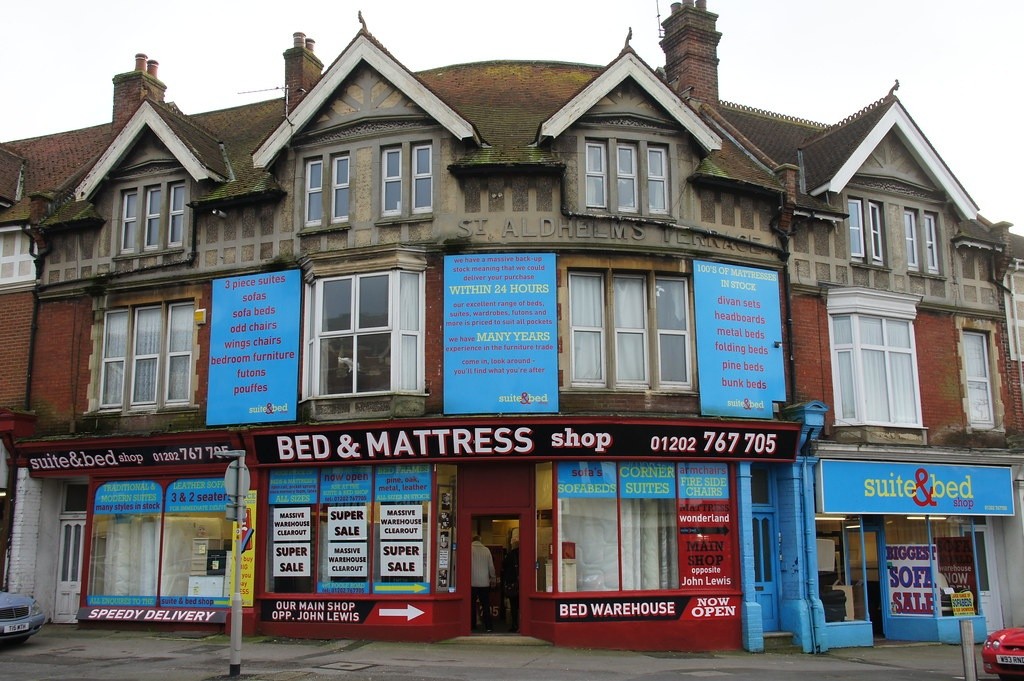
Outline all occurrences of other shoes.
[486,627,492,632]
[509,628,517,632]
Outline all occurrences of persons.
[471,535,497,633]
[442,493,450,510]
[501,548,520,632]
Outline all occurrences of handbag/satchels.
[504,583,519,597]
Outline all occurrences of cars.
[982,625,1024,681]
[0,591,45,646]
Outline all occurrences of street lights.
[214,449,243,676]
